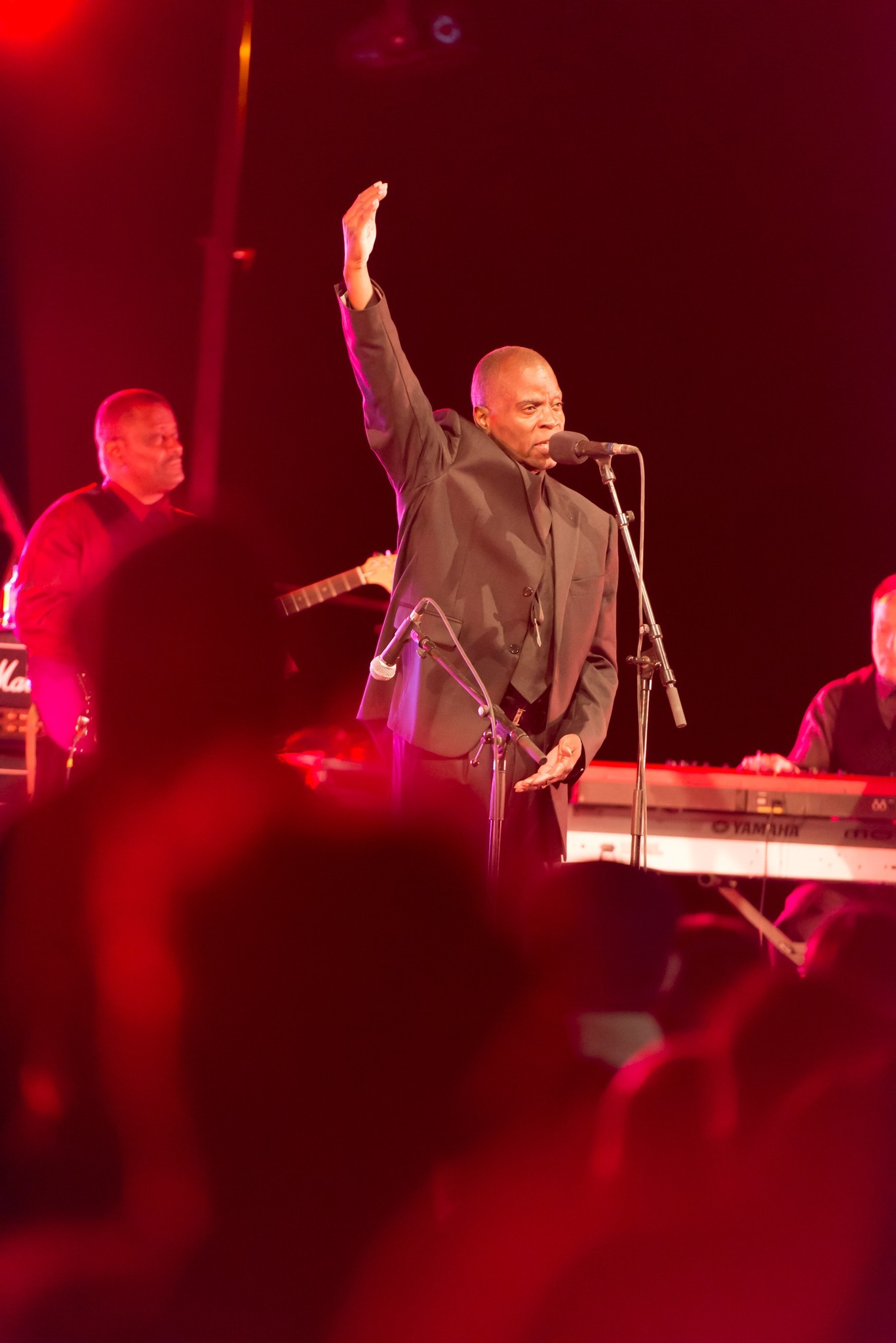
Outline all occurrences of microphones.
[370,598,428,681]
[549,430,637,465]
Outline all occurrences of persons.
[0,513,896,1343]
[335,180,619,865]
[5,390,194,805]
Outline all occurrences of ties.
[510,534,552,705]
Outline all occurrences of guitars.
[30,550,402,756]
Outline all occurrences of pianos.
[564,759,896,972]
